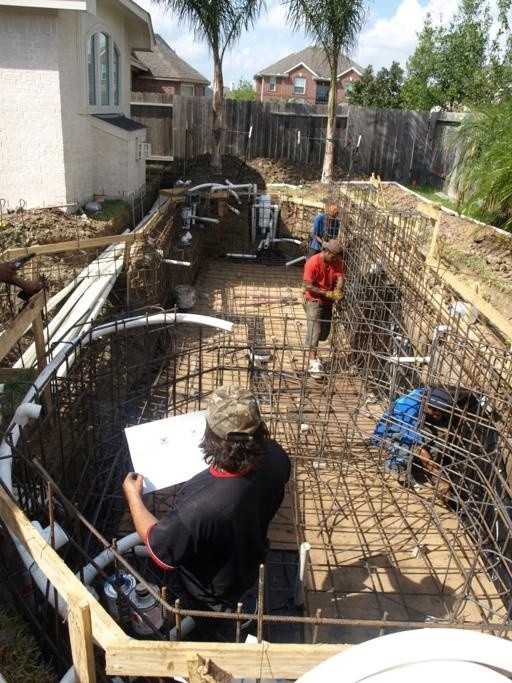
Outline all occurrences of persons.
[301,240,347,381]
[307,202,340,259]
[369,385,453,503]
[103,382,293,626]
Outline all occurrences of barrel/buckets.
[175,285,195,308]
[447,301,477,324]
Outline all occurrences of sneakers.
[309,356,327,379]
[430,389,456,413]
[389,462,408,484]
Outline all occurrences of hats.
[207,384,260,442]
[324,240,343,255]
[326,204,339,219]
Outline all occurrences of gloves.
[430,472,451,504]
[326,290,343,301]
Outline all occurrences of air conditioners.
[137,142,151,160]
